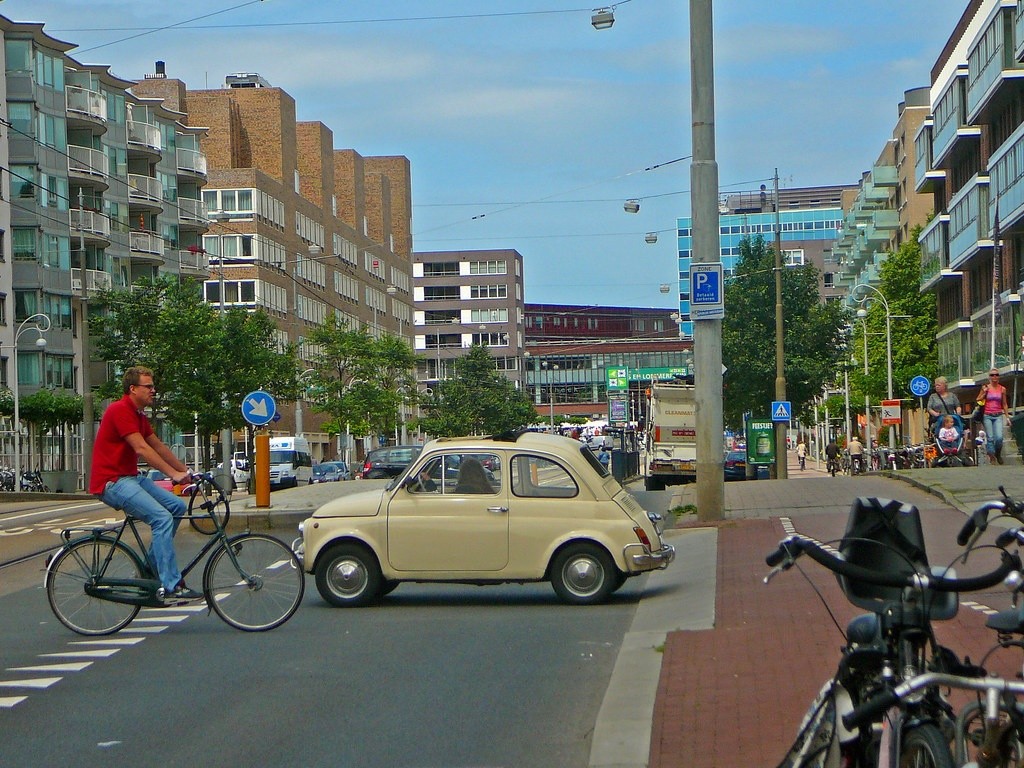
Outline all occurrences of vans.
[586,436,614,452]
[232,436,313,491]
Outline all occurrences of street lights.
[394,384,414,447]
[15,314,51,491]
[851,283,894,453]
[417,387,434,439]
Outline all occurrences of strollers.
[930,414,974,467]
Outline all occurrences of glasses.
[989,374,999,377]
[131,383,156,390]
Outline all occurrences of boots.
[988,454,997,465]
[994,448,1004,465]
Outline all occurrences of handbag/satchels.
[972,406,984,424]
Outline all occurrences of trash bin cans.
[629,451,640,476]
[611,451,628,478]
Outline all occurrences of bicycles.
[39,471,305,637]
[796,451,805,472]
[0,461,52,493]
[759,484,1024,768]
[829,442,936,477]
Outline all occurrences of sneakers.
[163,579,204,605]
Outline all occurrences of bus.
[362,445,496,481]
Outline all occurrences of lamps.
[670,313,682,325]
[679,332,694,370]
[591,7,616,31]
[660,284,670,294]
[503,334,510,340]
[524,351,531,358]
[308,245,321,257]
[554,365,559,370]
[479,324,486,330]
[542,360,548,366]
[624,199,640,214]
[645,232,657,243]
[387,285,397,295]
[452,318,461,324]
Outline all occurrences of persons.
[976,368,1012,465]
[795,441,807,465]
[597,447,610,469]
[939,415,959,454]
[847,436,863,476]
[422,458,494,493]
[826,439,840,473]
[976,430,985,447]
[927,376,961,467]
[88,365,204,605]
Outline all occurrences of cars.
[308,462,365,485]
[479,453,500,472]
[289,428,675,609]
[723,451,760,481]
[216,459,244,475]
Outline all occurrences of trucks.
[643,383,697,492]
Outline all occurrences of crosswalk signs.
[771,402,791,421]
